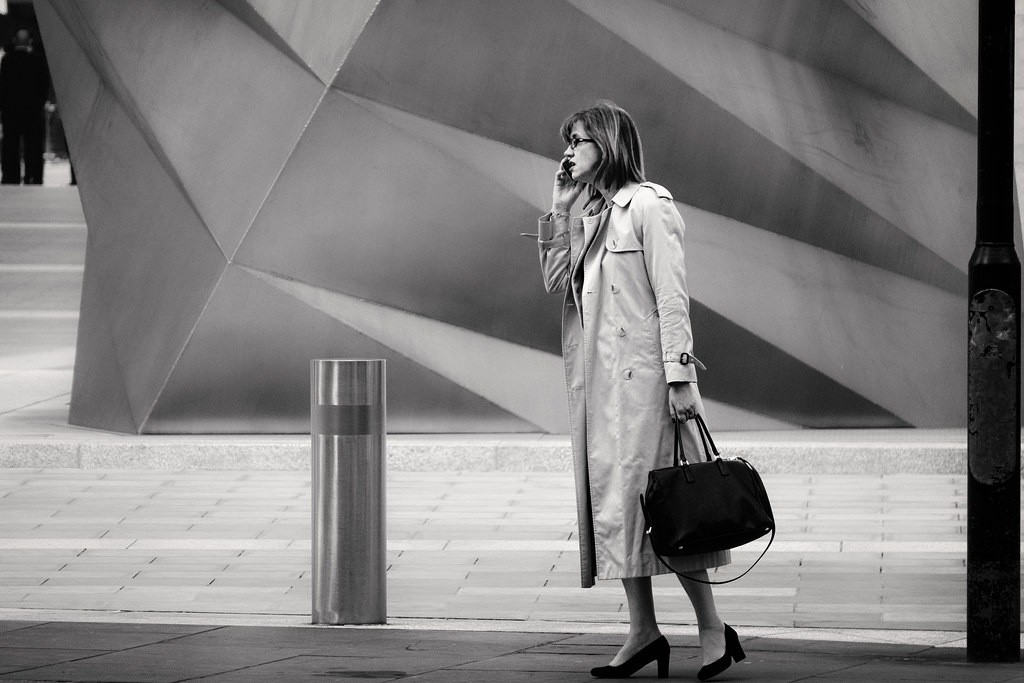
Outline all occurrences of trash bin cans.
[311,357,389,626]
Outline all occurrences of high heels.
[697,623,746,682]
[590,635,670,680]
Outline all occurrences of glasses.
[569,138,596,149]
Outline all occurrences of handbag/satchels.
[640,413,776,584]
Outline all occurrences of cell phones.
[561,159,574,181]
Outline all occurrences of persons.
[0,29,75,186]
[521,99,747,681]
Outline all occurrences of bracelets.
[549,209,569,219]
